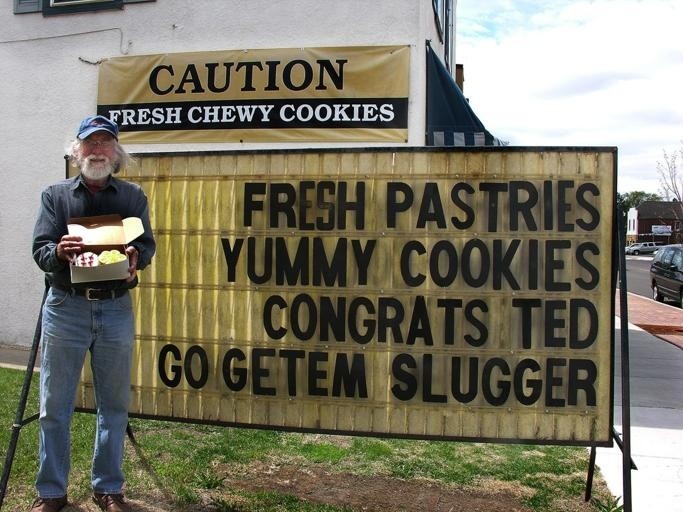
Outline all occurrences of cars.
[650,244,683,307]
[623,242,665,255]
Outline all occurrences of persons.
[28,113,154,512]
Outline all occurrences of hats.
[74,114,120,141]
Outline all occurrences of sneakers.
[28,493,69,512]
[92,491,134,512]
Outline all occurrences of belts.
[47,280,128,302]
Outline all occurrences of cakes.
[75,249,127,268]
